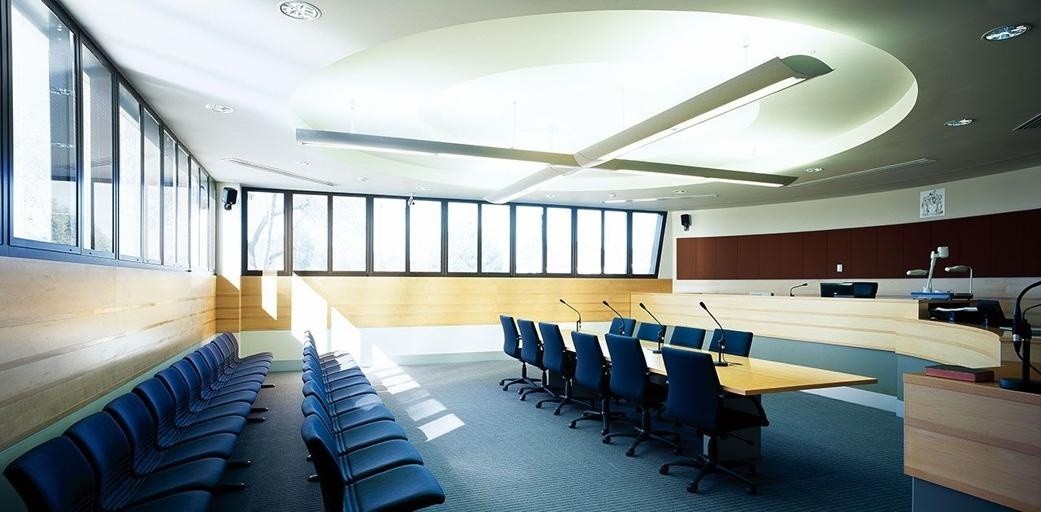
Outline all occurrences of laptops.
[821,283,854,297]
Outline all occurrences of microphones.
[1000,281,1041,392]
[699,302,728,366]
[790,283,807,296]
[602,300,624,336]
[639,303,665,354]
[560,299,581,332]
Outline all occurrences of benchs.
[1,329,274,512]
[301,329,447,512]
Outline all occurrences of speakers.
[681,214,689,226]
[222,187,237,204]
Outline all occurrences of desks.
[895,301,1041,512]
[519,329,878,468]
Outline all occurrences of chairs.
[636,323,667,343]
[708,329,753,357]
[670,326,707,350]
[499,316,543,391]
[517,319,565,401]
[601,334,684,457]
[535,323,595,416]
[659,347,769,496]
[567,332,641,437]
[608,318,636,337]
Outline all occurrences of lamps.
[294,52,836,209]
[922,242,951,293]
[944,262,976,298]
[903,267,934,294]
